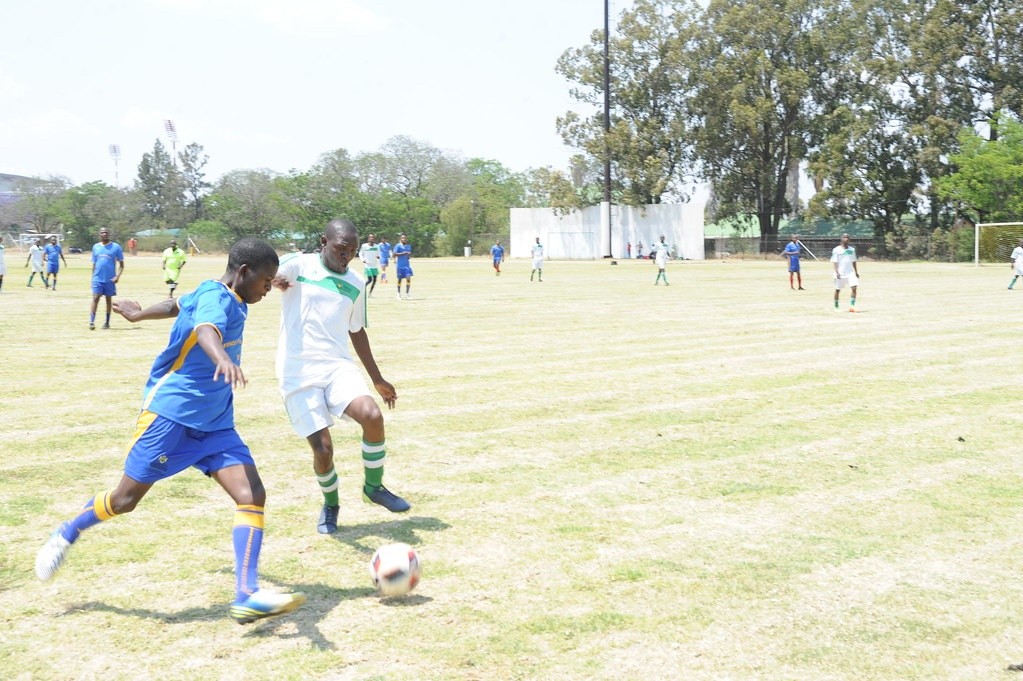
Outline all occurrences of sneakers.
[318,504,340,534]
[363,484,409,513]
[35,521,72,582]
[229,589,307,625]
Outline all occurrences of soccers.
[367,542,421,596]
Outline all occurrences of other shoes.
[90,325,95,330]
[102,324,109,329]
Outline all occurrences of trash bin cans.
[464,246,473,258]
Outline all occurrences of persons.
[0,237,5,292]
[830,234,860,312]
[128,238,136,255]
[491,240,504,275]
[626,242,631,257]
[393,234,413,299]
[37,239,307,625]
[654,235,671,286]
[25,239,52,287]
[163,240,187,298]
[89,228,124,330]
[635,241,642,254]
[531,237,543,282]
[379,237,393,283]
[1008,241,1023,289]
[271,219,411,533]
[42,237,67,290]
[359,233,380,298]
[785,236,806,290]
[649,244,656,258]
